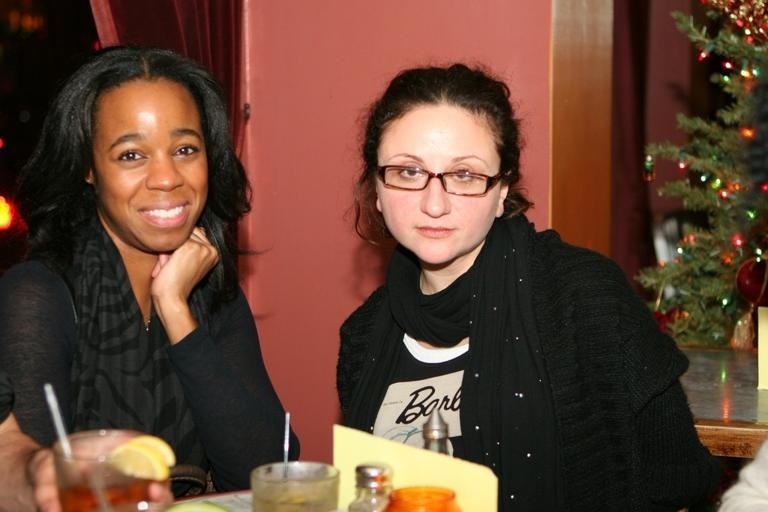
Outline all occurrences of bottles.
[422,406,449,455]
[347,463,394,511]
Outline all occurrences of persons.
[2,44,301,511]
[333,60,717,512]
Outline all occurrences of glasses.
[371,163,511,199]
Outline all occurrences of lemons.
[109,441,170,480]
[132,435,177,466]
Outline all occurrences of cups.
[51,428,147,511]
[250,461,341,511]
[387,485,462,511]
